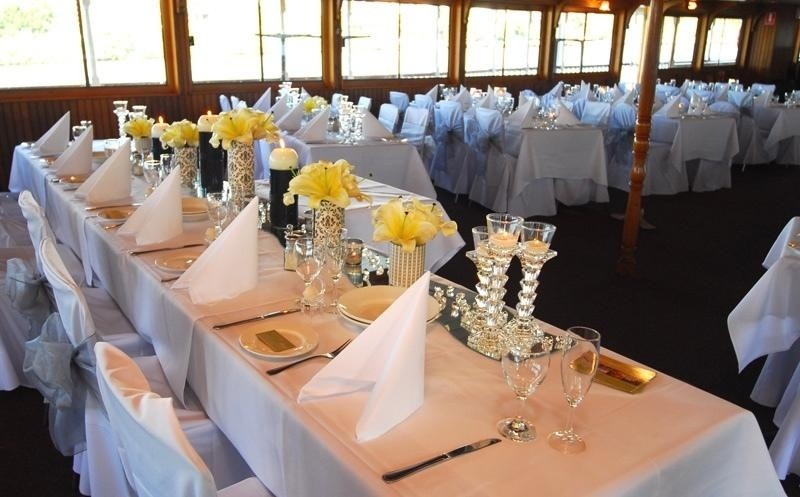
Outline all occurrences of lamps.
[597,1,611,12]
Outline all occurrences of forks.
[265,337,356,380]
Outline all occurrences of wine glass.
[292,236,326,308]
[496,335,551,442]
[275,93,365,146]
[226,184,245,218]
[325,228,346,307]
[203,190,229,236]
[534,107,559,131]
[443,86,515,115]
[547,327,601,452]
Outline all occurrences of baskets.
[389,241,426,287]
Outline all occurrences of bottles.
[285,234,300,272]
[340,237,364,265]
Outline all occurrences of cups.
[103,138,119,159]
[142,161,160,187]
[160,153,176,178]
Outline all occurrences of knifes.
[381,432,502,485]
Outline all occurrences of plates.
[337,285,441,331]
[181,197,209,222]
[96,209,132,224]
[154,254,202,275]
[238,323,319,358]
[60,173,86,187]
